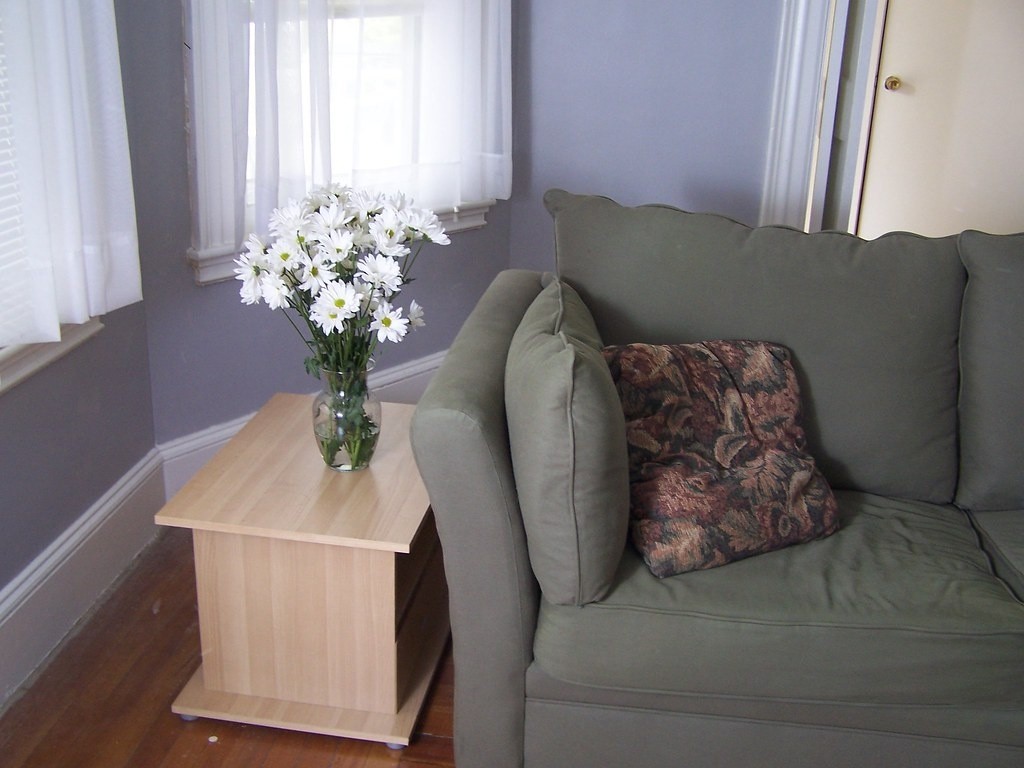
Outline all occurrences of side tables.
[154,392,451,748]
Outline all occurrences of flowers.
[232,177,452,471]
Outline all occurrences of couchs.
[410,188,1023,768]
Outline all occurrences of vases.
[312,358,382,471]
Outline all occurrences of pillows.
[599,337,843,579]
[504,276,631,606]
